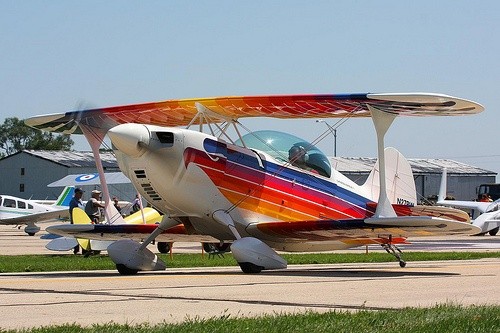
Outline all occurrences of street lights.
[316,118,336,157]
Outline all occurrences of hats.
[74,188,86,193]
[91,190,102,196]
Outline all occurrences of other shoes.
[73,252,83,255]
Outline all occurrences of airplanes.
[40,208,184,250]
[0,172,131,236]
[24,93,482,274]
[438,167,500,237]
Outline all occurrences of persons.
[479,193,493,215]
[113,194,141,219]
[69,187,86,254]
[83,190,105,258]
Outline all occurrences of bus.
[476,183,500,202]
